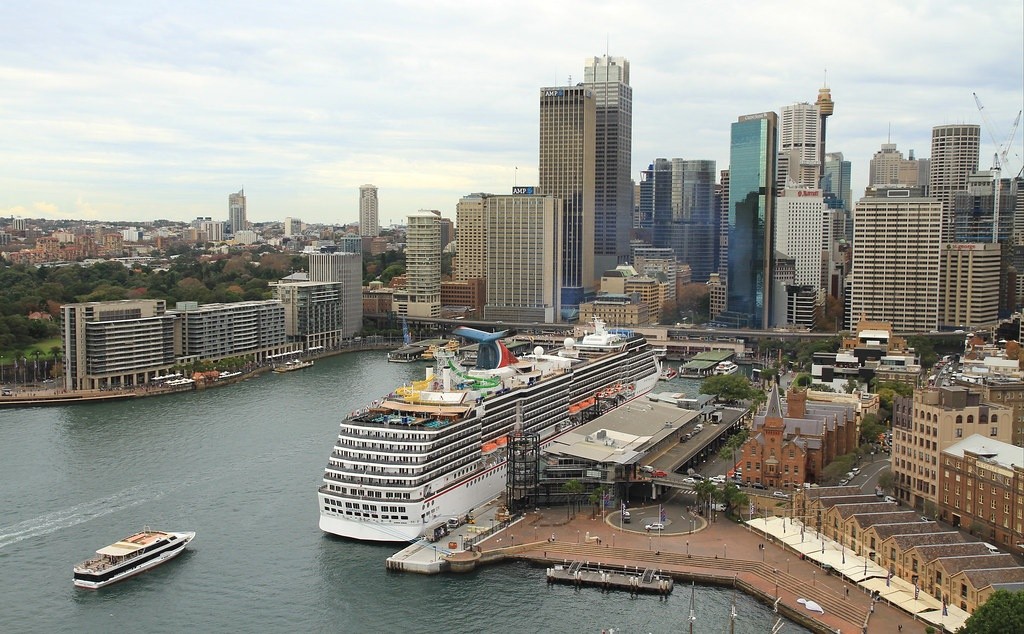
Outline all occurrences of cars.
[678,421,706,444]
[839,467,861,486]
[876,490,898,506]
[468,515,476,524]
[712,503,727,513]
[683,465,748,490]
[752,483,768,490]
[921,516,931,522]
[639,465,654,473]
[650,469,668,478]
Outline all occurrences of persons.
[511,533,764,560]
[846,588,849,595]
[687,504,702,516]
[897,624,902,632]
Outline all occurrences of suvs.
[645,523,664,531]
[772,491,788,498]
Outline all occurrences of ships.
[317,315,662,543]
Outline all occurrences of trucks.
[711,411,722,425]
[624,512,631,522]
[447,513,467,529]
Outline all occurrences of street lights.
[490,518,494,534]
[724,543,728,558]
[649,537,652,550]
[577,529,580,544]
[844,585,846,602]
[433,545,437,562]
[458,534,463,551]
[612,533,616,548]
[762,547,765,563]
[510,534,515,547]
[534,526,538,542]
[686,540,689,554]
[787,559,790,575]
[813,570,817,587]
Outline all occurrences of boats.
[494,437,510,448]
[713,360,739,377]
[72,528,196,590]
[479,443,497,456]
[588,397,599,406]
[612,384,625,391]
[596,393,605,400]
[659,369,678,382]
[602,388,615,396]
[566,406,583,416]
[577,402,590,410]
